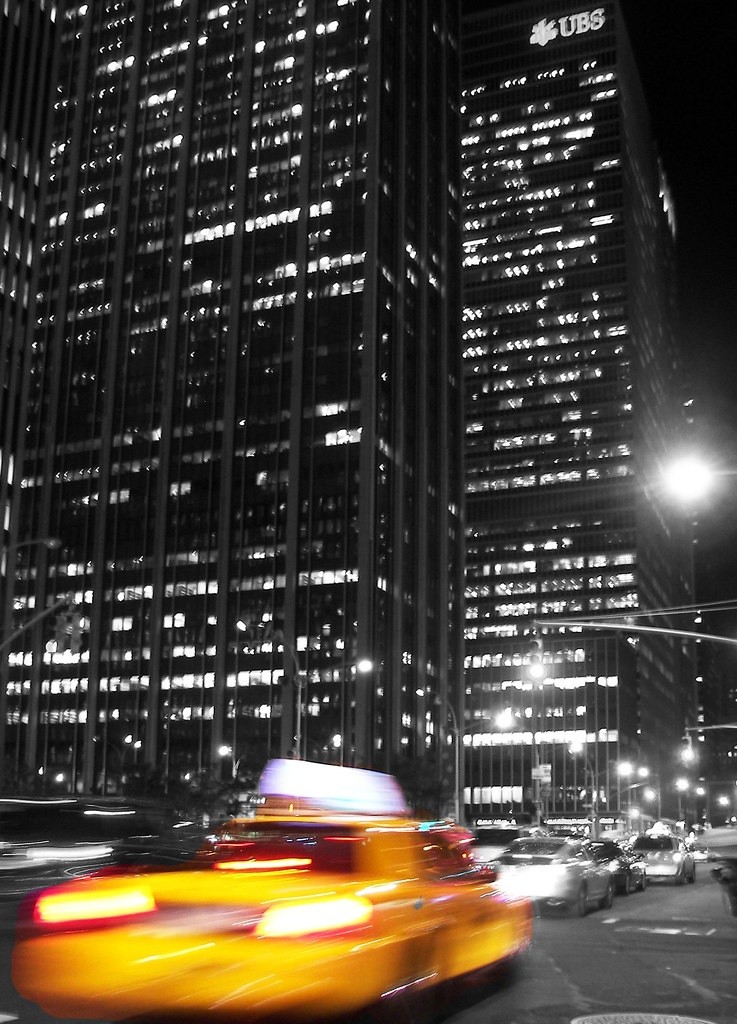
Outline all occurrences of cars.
[584,841,649,896]
[629,820,696,885]
[1,754,536,1024]
[688,841,708,862]
[490,834,616,918]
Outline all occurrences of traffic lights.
[697,780,706,795]
[681,740,696,762]
[71,613,91,654]
[720,793,728,806]
[530,637,545,680]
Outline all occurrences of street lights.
[417,687,517,824]
[664,449,717,830]
[238,620,374,760]
[570,741,633,840]
